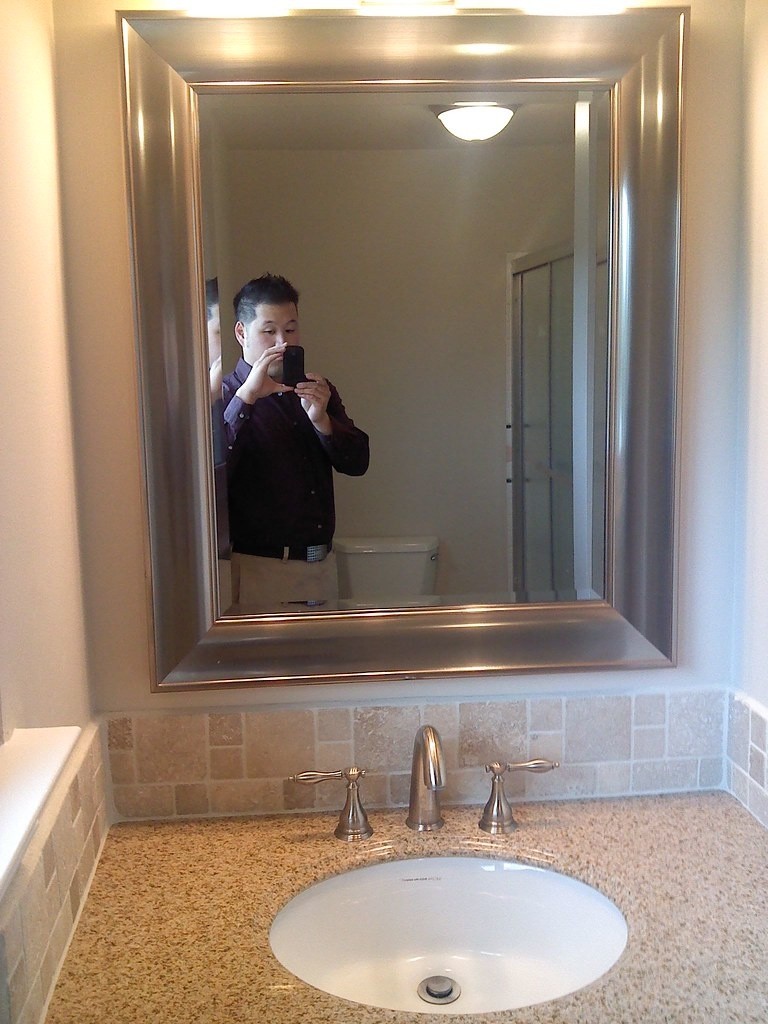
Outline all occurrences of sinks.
[269,841,631,1018]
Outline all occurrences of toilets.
[330,535,442,608]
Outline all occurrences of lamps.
[427,103,516,140]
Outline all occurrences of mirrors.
[117,5,691,693]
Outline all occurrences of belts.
[232,541,332,562]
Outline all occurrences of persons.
[222,271,370,610]
[204,276,222,466]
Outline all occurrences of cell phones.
[282,346,305,387]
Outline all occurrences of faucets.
[404,722,448,833]
